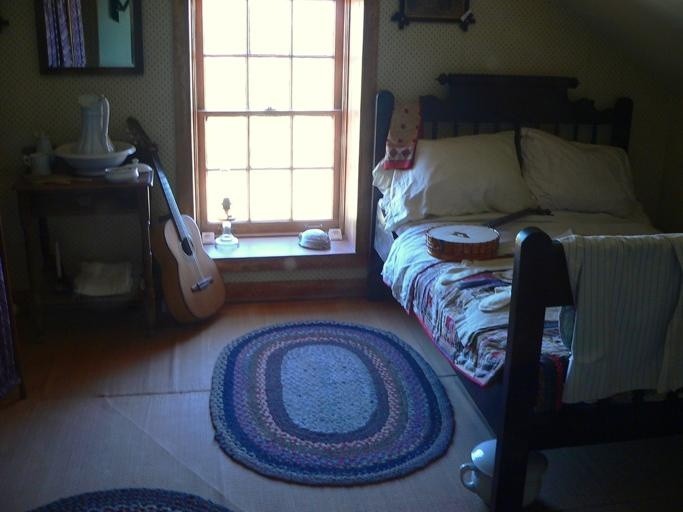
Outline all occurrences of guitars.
[125,116,227,324]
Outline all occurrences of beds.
[372,71,683,510]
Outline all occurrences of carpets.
[30,487,232,512]
[209,319,455,487]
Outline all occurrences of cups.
[31,154,49,174]
[460,440,548,512]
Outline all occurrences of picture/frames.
[391,1,473,31]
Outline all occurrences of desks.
[14,164,154,343]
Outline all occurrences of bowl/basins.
[55,140,136,177]
[105,167,138,182]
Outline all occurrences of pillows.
[372,130,539,234]
[520,125,638,218]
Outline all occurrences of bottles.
[215,222,239,252]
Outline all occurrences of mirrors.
[34,0,144,75]
